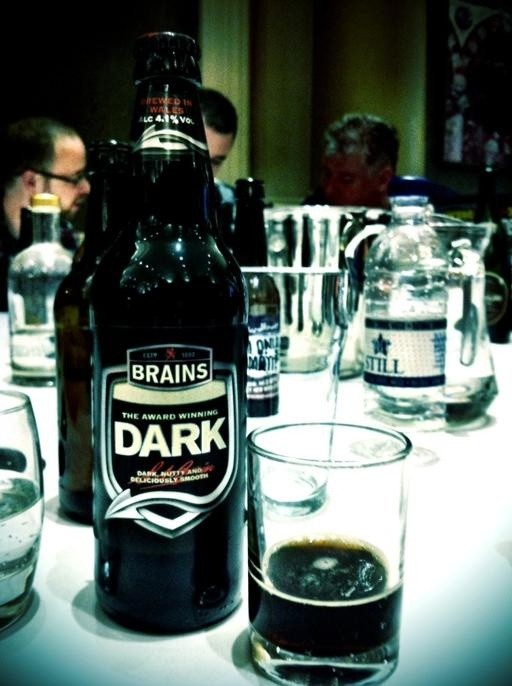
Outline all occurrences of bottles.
[91,34,247,641]
[365,175,446,419]
[5,194,70,389]
[52,140,133,529]
[230,174,282,419]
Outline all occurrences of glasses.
[32,169,84,185]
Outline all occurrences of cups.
[237,265,341,518]
[0,388,43,625]
[240,423,402,686]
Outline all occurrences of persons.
[1,117,92,313]
[294,109,478,214]
[198,85,240,230]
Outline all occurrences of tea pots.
[333,222,500,431]
